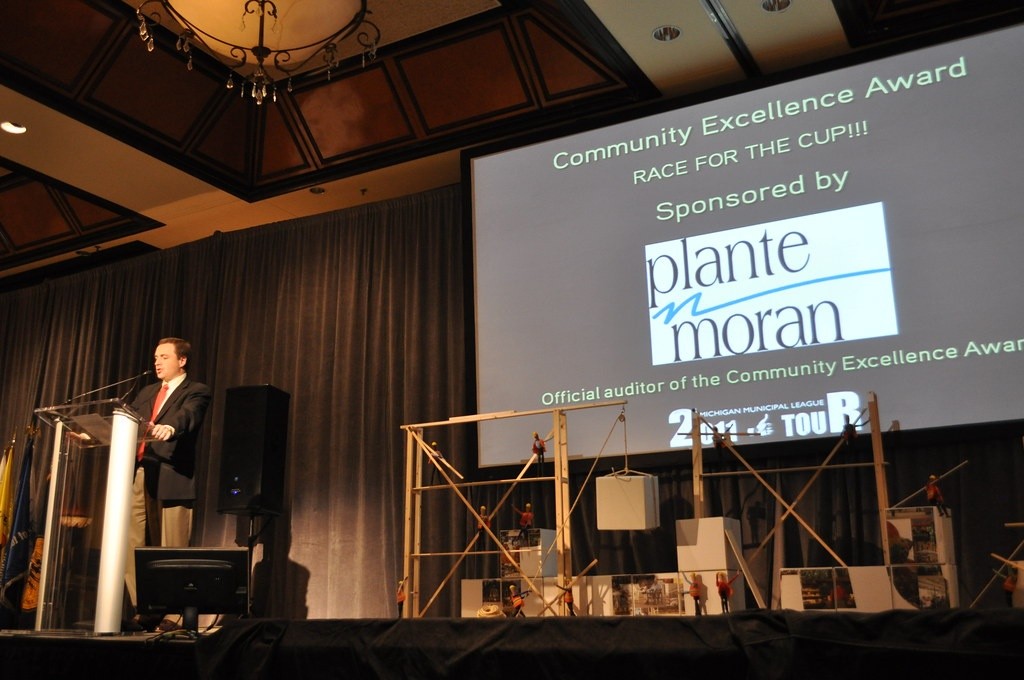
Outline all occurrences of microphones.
[58,369,153,406]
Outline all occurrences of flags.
[0,435,49,613]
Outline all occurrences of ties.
[137,385,168,461]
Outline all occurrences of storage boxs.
[780,567,837,613]
[594,476,661,531]
[590,574,634,617]
[884,506,956,564]
[632,572,681,616]
[544,575,593,616]
[890,564,960,611]
[502,578,544,617]
[498,530,558,577]
[674,517,742,572]
[680,570,745,615]
[460,578,502,619]
[836,565,893,612]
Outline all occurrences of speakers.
[217,383,291,515]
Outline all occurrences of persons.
[490,582,498,601]
[531,432,546,477]
[830,570,847,609]
[397,577,408,618]
[564,578,577,616]
[427,442,443,485]
[712,427,724,472]
[511,503,533,538]
[843,415,859,462]
[689,573,702,616]
[924,475,951,518]
[650,576,661,589]
[715,570,740,614]
[477,506,491,551]
[509,585,532,616]
[79,337,212,632]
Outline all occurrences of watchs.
[165,426,172,431]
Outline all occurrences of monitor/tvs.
[134,547,250,639]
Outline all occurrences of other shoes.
[155,619,182,634]
[126,613,154,632]
